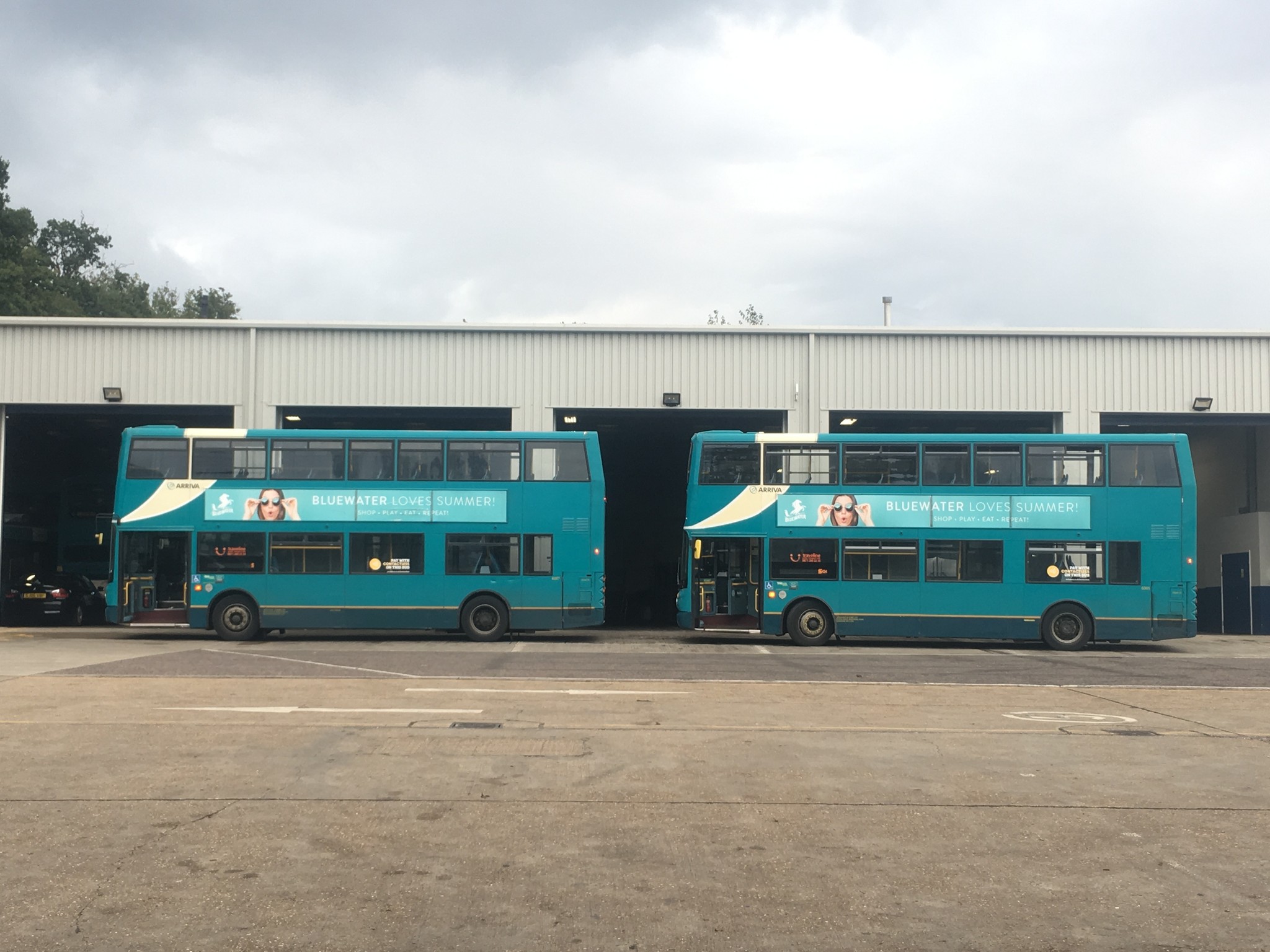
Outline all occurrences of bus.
[93,425,605,642]
[675,431,1197,650]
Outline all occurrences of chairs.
[162,466,491,481]
[703,472,1144,485]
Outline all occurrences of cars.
[4,570,107,627]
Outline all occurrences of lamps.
[663,393,680,406]
[1193,398,1213,411]
[103,387,122,402]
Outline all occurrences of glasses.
[261,498,280,505]
[834,503,854,511]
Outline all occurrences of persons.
[242,488,302,522]
[817,494,874,527]
